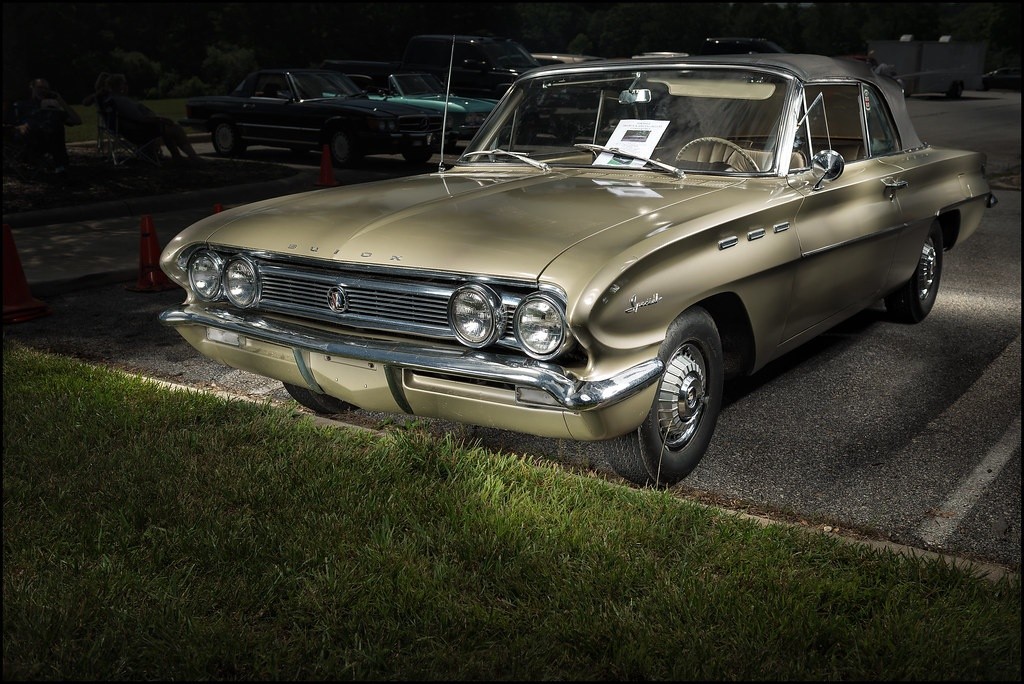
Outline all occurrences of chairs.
[2,92,165,170]
[673,133,867,173]
[263,83,282,99]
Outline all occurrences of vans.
[699,36,788,60]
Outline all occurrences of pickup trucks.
[319,33,602,146]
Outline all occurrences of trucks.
[864,37,986,101]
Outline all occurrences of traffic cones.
[211,203,225,215]
[312,143,340,187]
[125,214,178,294]
[0,223,51,325]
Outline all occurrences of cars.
[159,53,997,490]
[175,67,453,169]
[322,70,502,154]
[982,66,1020,96]
